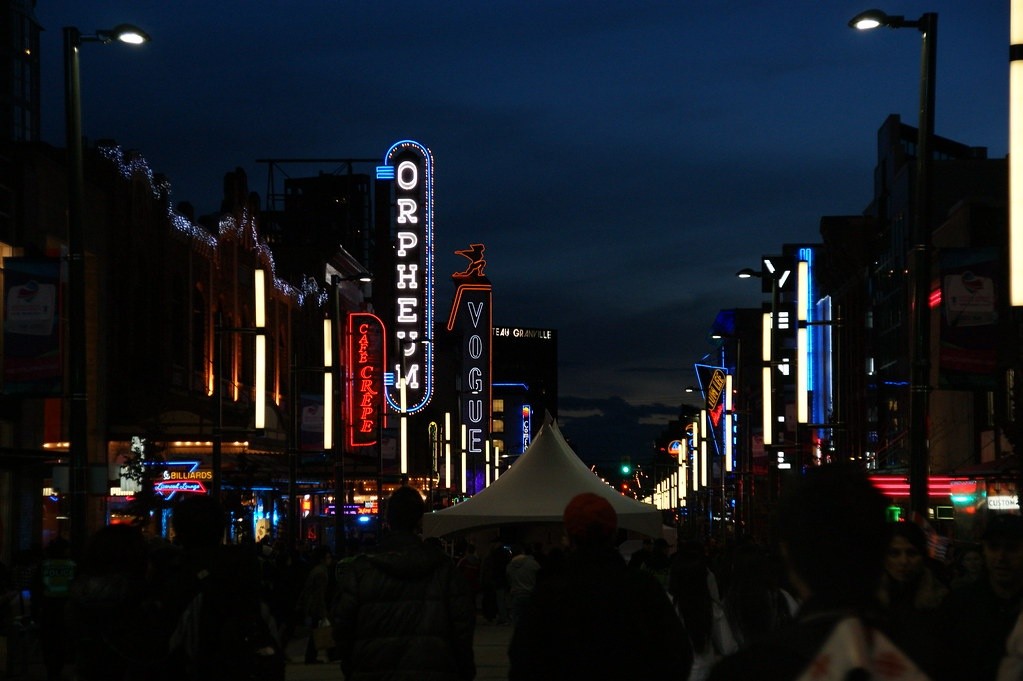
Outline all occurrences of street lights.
[61,23,154,536]
[710,332,742,476]
[846,7,941,519]
[683,386,712,491]
[331,271,374,564]
[735,268,779,476]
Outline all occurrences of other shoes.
[496,620,510,626]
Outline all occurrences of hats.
[564,493,617,537]
[976,512,1023,541]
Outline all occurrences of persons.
[325,487,478,681]
[506,492,694,680]
[0,486,1023,681]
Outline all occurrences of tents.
[422,416,661,541]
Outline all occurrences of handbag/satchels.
[313,620,329,653]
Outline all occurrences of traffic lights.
[620,454,632,476]
